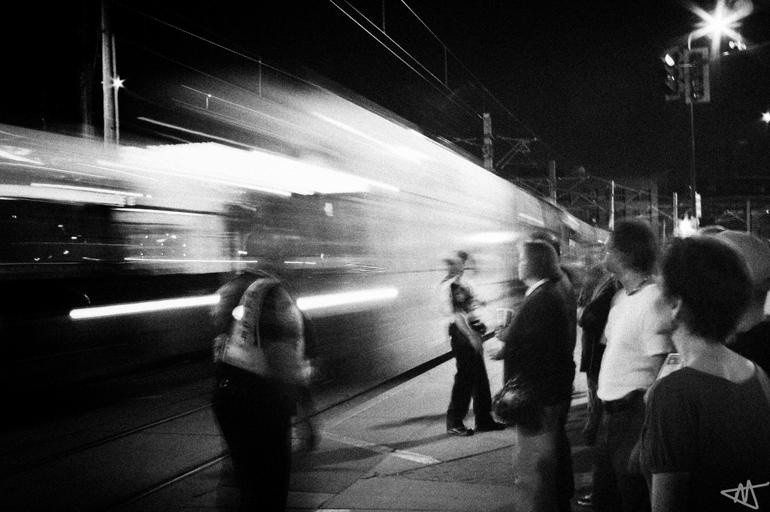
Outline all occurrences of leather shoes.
[475,422,507,432]
[445,422,475,437]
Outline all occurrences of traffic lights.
[660,45,711,105]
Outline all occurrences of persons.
[439,251,509,435]
[208,225,315,511]
[273,283,325,462]
